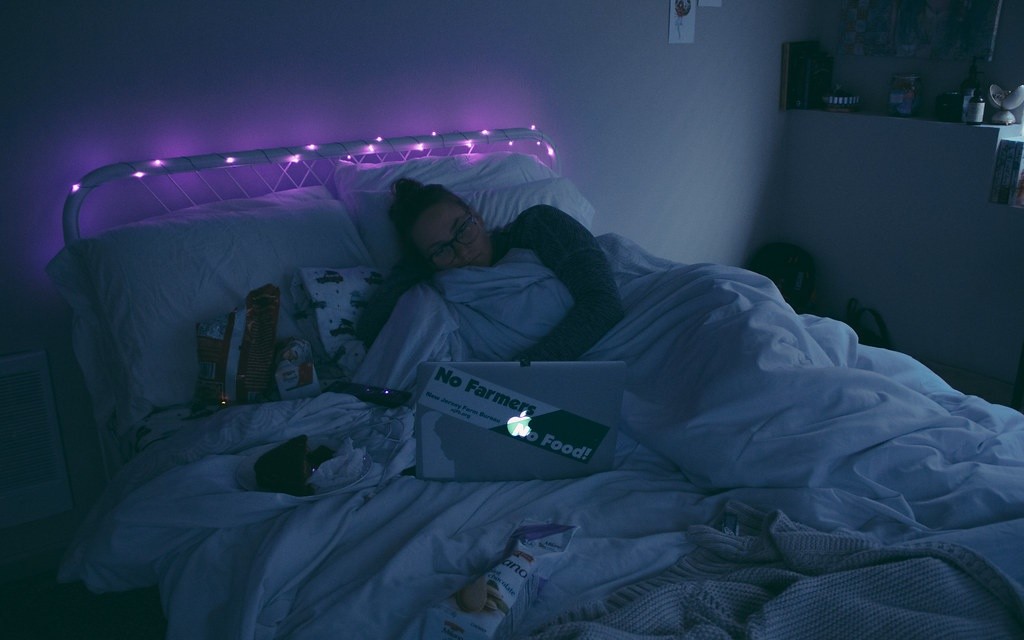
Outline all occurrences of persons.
[357,178,624,360]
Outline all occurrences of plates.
[234,437,373,498]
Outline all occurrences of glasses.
[429,213,480,269]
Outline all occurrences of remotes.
[322,380,413,408]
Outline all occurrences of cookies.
[458,574,503,612]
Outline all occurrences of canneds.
[887,73,920,117]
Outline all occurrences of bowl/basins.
[822,95,861,112]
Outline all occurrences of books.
[779,41,833,111]
[991,135,1024,207]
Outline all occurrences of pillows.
[43,152,595,434]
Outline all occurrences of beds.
[61,125,1024,640]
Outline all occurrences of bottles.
[935,91,963,122]
[847,4,857,35]
[966,96,985,125]
[856,18,865,56]
[887,74,921,117]
[963,89,974,121]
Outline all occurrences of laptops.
[414,361,627,481]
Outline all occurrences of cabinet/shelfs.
[785,107,1024,404]
